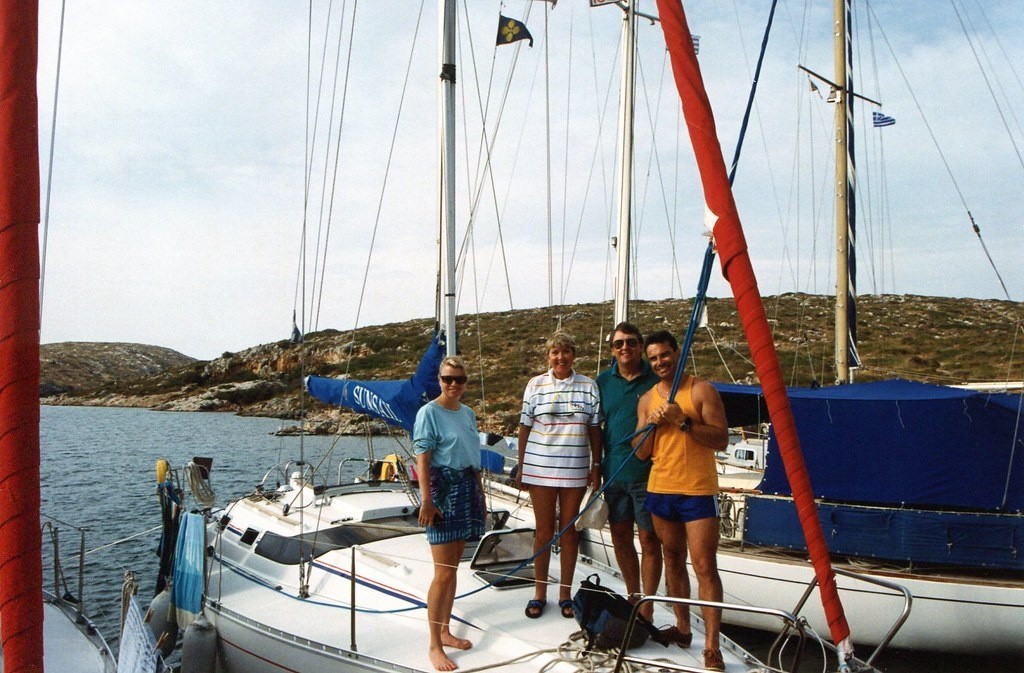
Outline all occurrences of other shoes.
[656,626,693,648]
[702,648,724,671]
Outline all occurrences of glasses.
[440,375,467,385]
[610,338,639,349]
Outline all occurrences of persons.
[413,356,488,671]
[595,322,661,623]
[631,331,729,672]
[515,332,603,618]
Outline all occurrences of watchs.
[679,417,692,433]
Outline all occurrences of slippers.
[559,600,575,618]
[525,600,546,619]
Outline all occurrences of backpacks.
[571,574,648,652]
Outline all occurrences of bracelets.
[592,463,600,467]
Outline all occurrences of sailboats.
[171,0,912,673]
[0,0,174,673]
[481,1,1024,656]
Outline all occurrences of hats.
[575,483,609,531]
